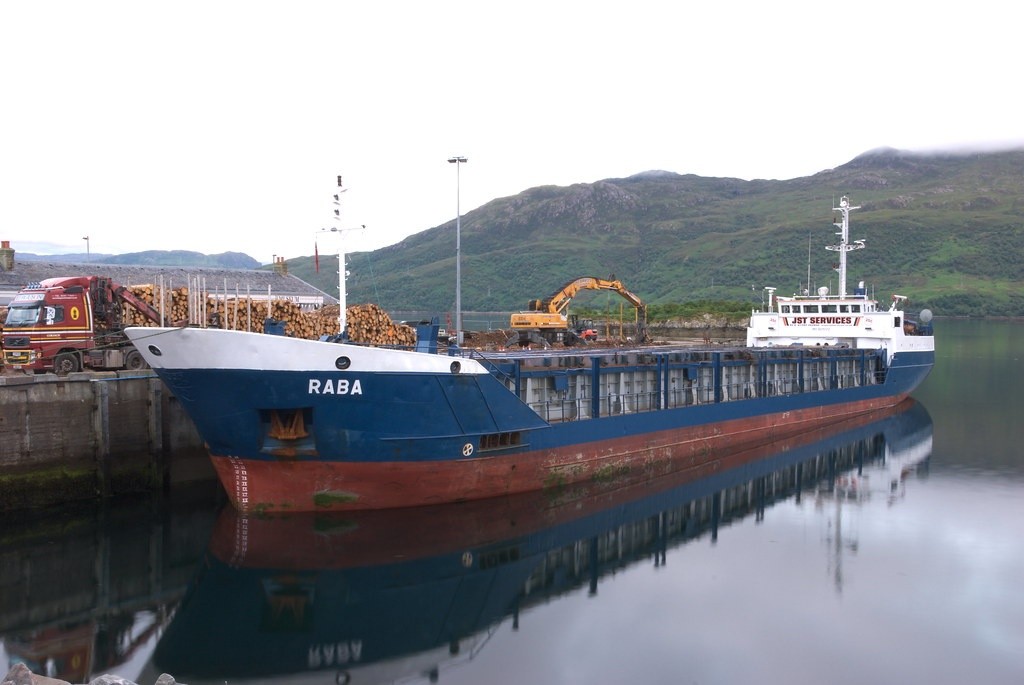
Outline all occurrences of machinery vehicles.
[505,275,653,348]
[568,314,598,341]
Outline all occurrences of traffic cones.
[528,345,531,350]
[544,344,547,350]
[498,345,505,352]
[522,345,525,351]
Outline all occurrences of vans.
[438,329,446,337]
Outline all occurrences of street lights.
[446,156,468,348]
[272,254,276,274]
[83,237,90,276]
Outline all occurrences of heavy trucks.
[0,276,222,377]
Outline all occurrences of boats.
[130,396,931,685]
[123,193,938,517]
